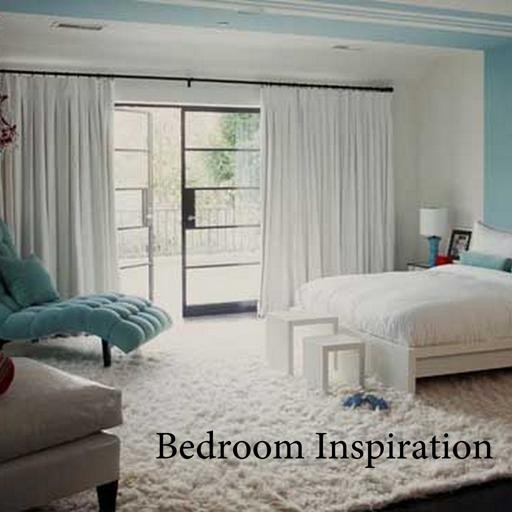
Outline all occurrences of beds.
[296,263,512,397]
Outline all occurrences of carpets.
[1,312,512,512]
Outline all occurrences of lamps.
[419,207,446,267]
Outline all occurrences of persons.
[453,235,467,259]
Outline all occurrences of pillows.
[0,254,61,308]
[461,219,512,272]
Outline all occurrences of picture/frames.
[447,229,471,260]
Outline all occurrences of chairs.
[0,218,175,367]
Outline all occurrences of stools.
[262,308,366,395]
[0,354,122,512]
